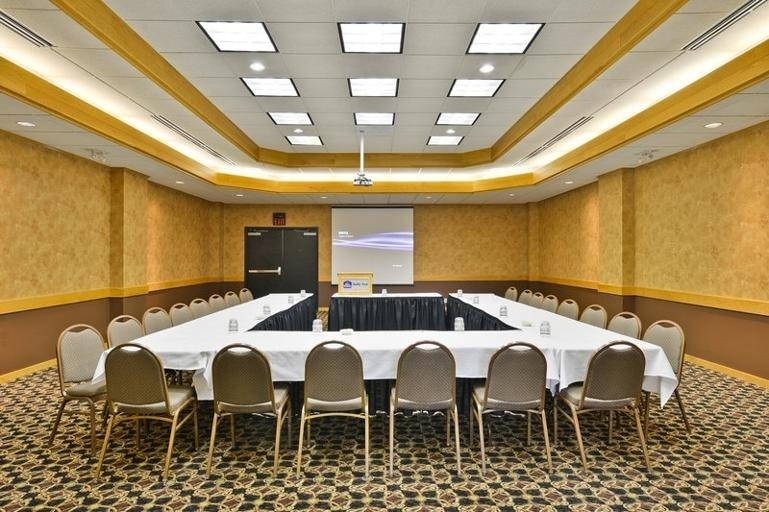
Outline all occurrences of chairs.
[542,295,557,314]
[605,309,642,342]
[171,303,194,326]
[208,293,227,311]
[98,347,202,479]
[189,298,212,319]
[643,318,692,437]
[239,287,253,304]
[505,286,518,301]
[518,289,533,304]
[582,305,609,331]
[385,339,467,478]
[105,313,146,347]
[207,342,292,478]
[225,290,240,309]
[558,300,580,320]
[143,306,172,335]
[50,323,115,449]
[530,291,544,308]
[563,341,651,471]
[298,339,370,483]
[475,340,553,474]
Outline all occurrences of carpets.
[0,361,769,510]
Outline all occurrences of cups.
[453,318,465,336]
[229,319,238,332]
[312,319,324,340]
[540,321,552,336]
[340,328,354,347]
[287,295,294,304]
[472,296,479,304]
[457,289,463,297]
[262,304,271,315]
[499,304,508,317]
[382,288,387,296]
[300,289,306,297]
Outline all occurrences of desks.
[329,293,446,333]
[100,292,675,471]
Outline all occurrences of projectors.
[352,177,371,186]
